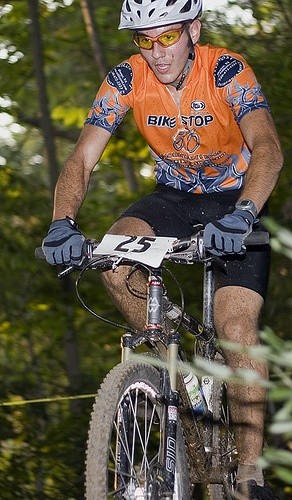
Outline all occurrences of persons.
[39,1,284,499]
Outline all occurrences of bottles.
[179,364,208,416]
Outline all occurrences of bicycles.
[36,231,269,500]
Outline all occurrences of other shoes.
[230,472,278,500]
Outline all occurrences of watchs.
[234,198,260,225]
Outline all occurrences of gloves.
[45,216,108,272]
[205,210,256,259]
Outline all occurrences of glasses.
[132,23,189,50]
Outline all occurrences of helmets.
[118,0,204,31]
